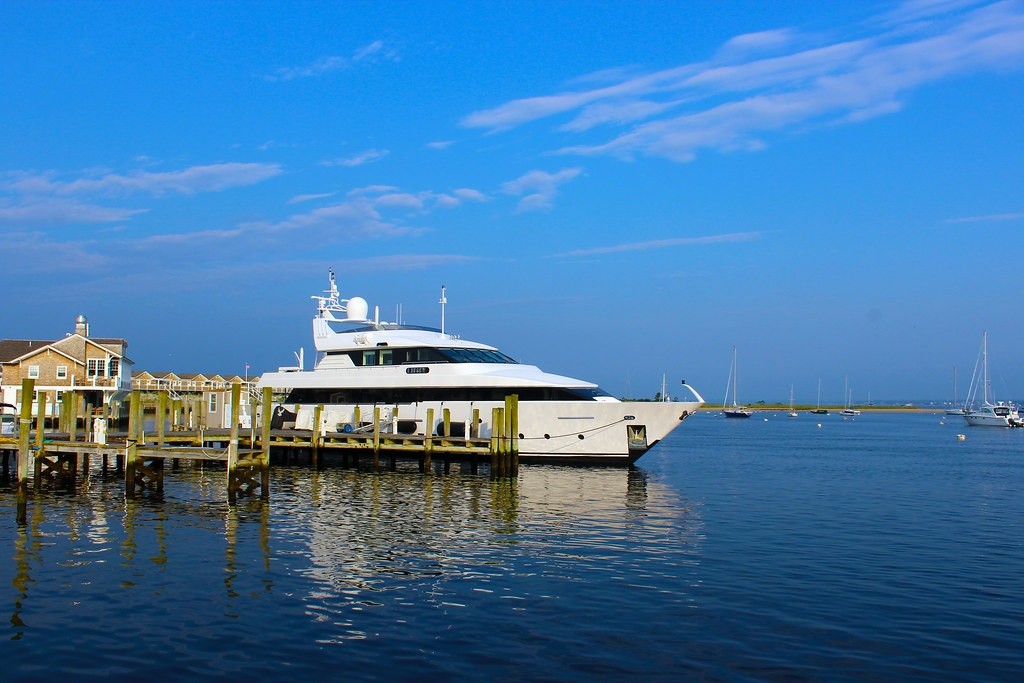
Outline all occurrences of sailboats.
[945,364,974,414]
[959,329,1024,427]
[787,383,800,417]
[719,347,754,419]
[839,376,861,417]
[809,375,830,415]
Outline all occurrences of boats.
[251,267,707,459]
